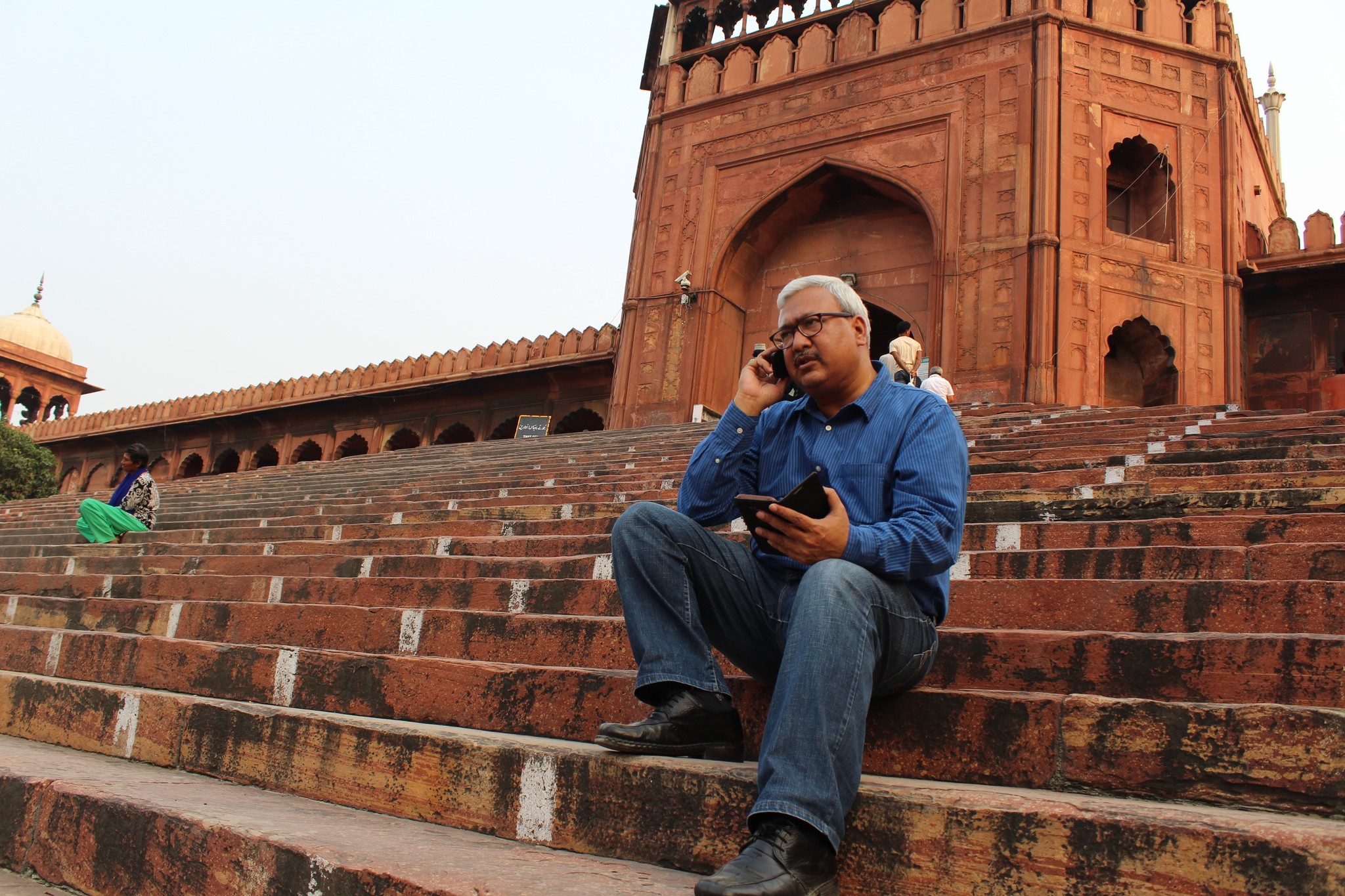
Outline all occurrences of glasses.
[770,311,854,349]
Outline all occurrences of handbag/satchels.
[908,373,915,387]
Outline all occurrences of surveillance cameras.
[674,270,692,283]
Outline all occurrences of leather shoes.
[693,813,839,896]
[595,689,744,762]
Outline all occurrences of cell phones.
[738,495,788,557]
[771,351,789,379]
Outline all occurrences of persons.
[920,365,954,404]
[889,321,922,387]
[879,353,895,382]
[595,274,972,896]
